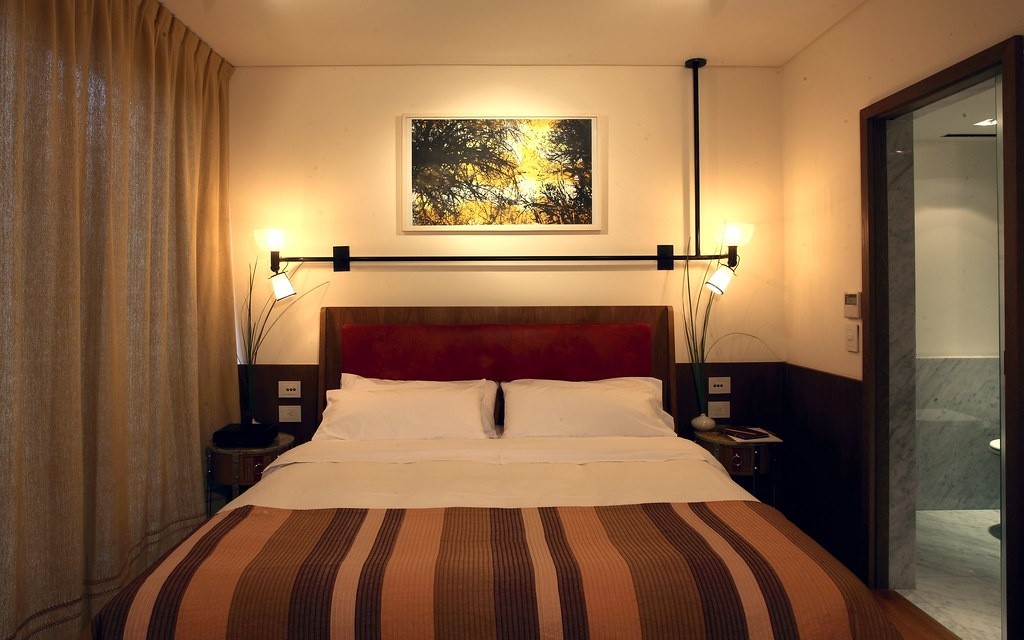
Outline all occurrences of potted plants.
[234,255,333,424]
[679,236,776,431]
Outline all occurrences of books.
[726,427,783,443]
[717,425,770,439]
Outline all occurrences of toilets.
[916,407,1001,460]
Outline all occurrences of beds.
[90,431,908,640]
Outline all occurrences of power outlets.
[708,401,730,418]
[277,380,302,399]
[708,377,732,395]
[278,405,302,423]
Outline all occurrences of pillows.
[338,371,502,439]
[499,376,679,440]
[309,378,491,440]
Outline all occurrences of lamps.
[268,268,297,302]
[704,263,735,296]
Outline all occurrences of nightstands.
[695,425,786,514]
[204,434,296,518]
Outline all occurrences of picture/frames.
[399,110,605,232]
